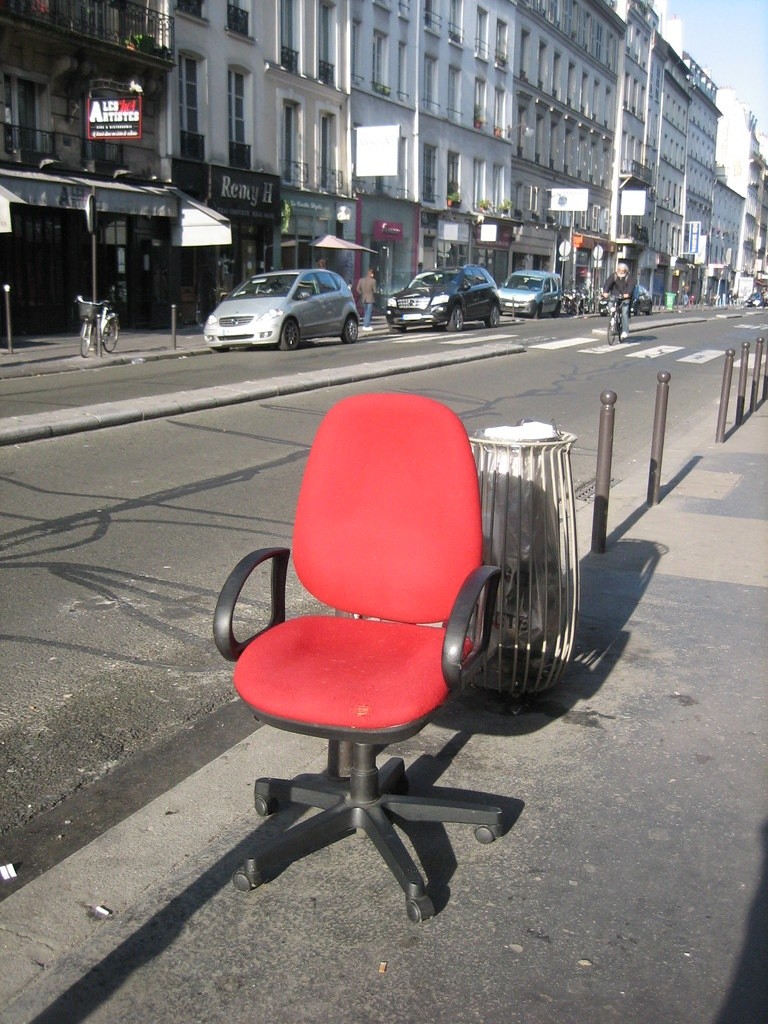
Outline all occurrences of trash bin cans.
[473,415,581,719]
[665,292,677,310]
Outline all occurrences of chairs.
[212,391,506,926]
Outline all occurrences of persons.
[356,268,377,331]
[683,286,690,307]
[602,262,633,339]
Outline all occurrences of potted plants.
[480,199,493,211]
[499,201,512,214]
[125,33,156,55]
[447,193,462,208]
[474,105,484,128]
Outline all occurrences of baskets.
[78,302,99,322]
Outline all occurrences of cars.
[599,284,653,317]
[745,293,765,307]
[497,269,562,320]
[202,269,360,353]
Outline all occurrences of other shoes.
[609,318,616,326]
[362,325,373,332]
[621,332,627,339]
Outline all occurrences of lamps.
[39,159,61,169]
[508,122,535,138]
[114,170,132,180]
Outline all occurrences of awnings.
[308,233,380,254]
[0,167,179,218]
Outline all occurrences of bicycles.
[599,294,626,345]
[74,296,120,358]
[562,288,596,314]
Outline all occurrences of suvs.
[384,263,503,334]
